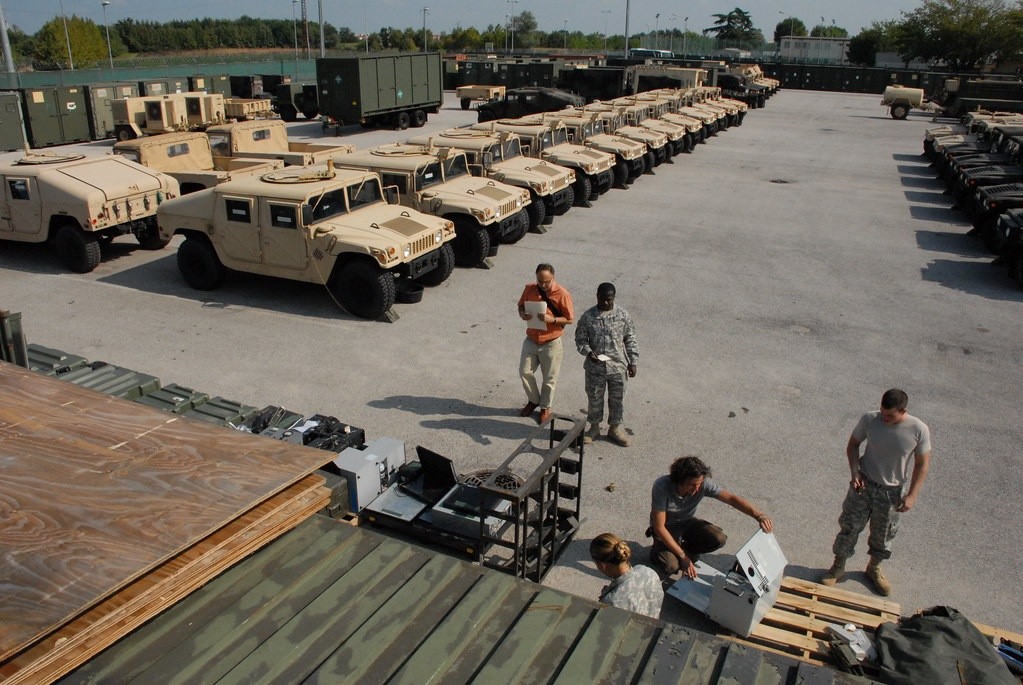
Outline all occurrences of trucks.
[205,121,356,166]
[104,132,285,195]
[633,60,780,109]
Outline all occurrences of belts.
[858,470,902,493]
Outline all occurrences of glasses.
[536,277,552,286]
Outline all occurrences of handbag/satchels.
[559,311,567,329]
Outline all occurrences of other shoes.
[650,547,660,566]
[583,427,600,444]
[681,543,698,562]
[521,400,539,417]
[607,426,630,447]
[539,409,549,425]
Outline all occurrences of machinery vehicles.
[924,105,1023,291]
[470,118,616,207]
[0,141,180,274]
[155,158,455,321]
[520,86,748,188]
[402,129,576,233]
[318,136,529,269]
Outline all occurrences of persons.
[589,533,664,622]
[575,282,639,447]
[822,388,931,594]
[643,456,772,580]
[517,264,574,424]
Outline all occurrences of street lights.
[602,11,612,54]
[779,11,794,64]
[668,18,676,52]
[507,0,518,53]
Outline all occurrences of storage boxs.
[664,524,788,638]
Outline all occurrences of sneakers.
[866,564,891,596]
[821,562,846,587]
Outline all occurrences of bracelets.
[756,513,764,521]
[553,317,556,324]
